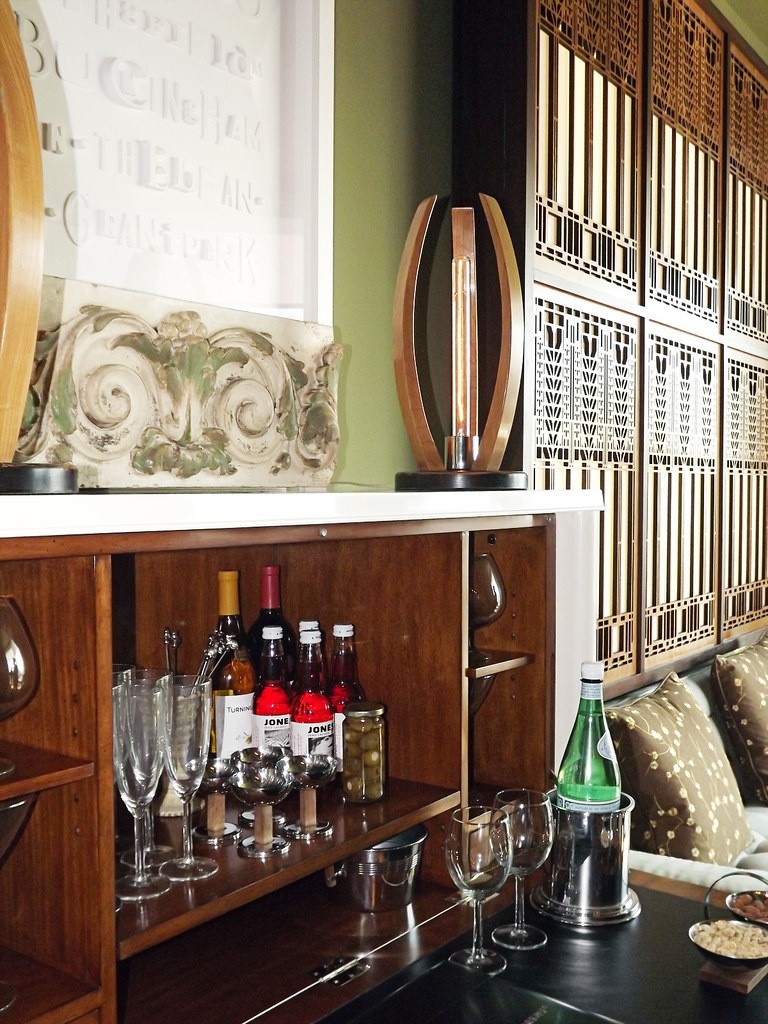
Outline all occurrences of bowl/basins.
[726,890,767,928]
[690,919,767,971]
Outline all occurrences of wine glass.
[465,552,506,665]
[112,661,219,907]
[2,799,37,1011]
[449,787,550,983]
[197,747,337,860]
[0,594,43,777]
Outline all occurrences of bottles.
[559,662,622,810]
[203,565,365,799]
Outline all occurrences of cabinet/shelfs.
[0,485,610,1024]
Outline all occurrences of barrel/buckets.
[531,790,642,929]
[332,827,429,912]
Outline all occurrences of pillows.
[599,670,757,869]
[709,628,767,803]
